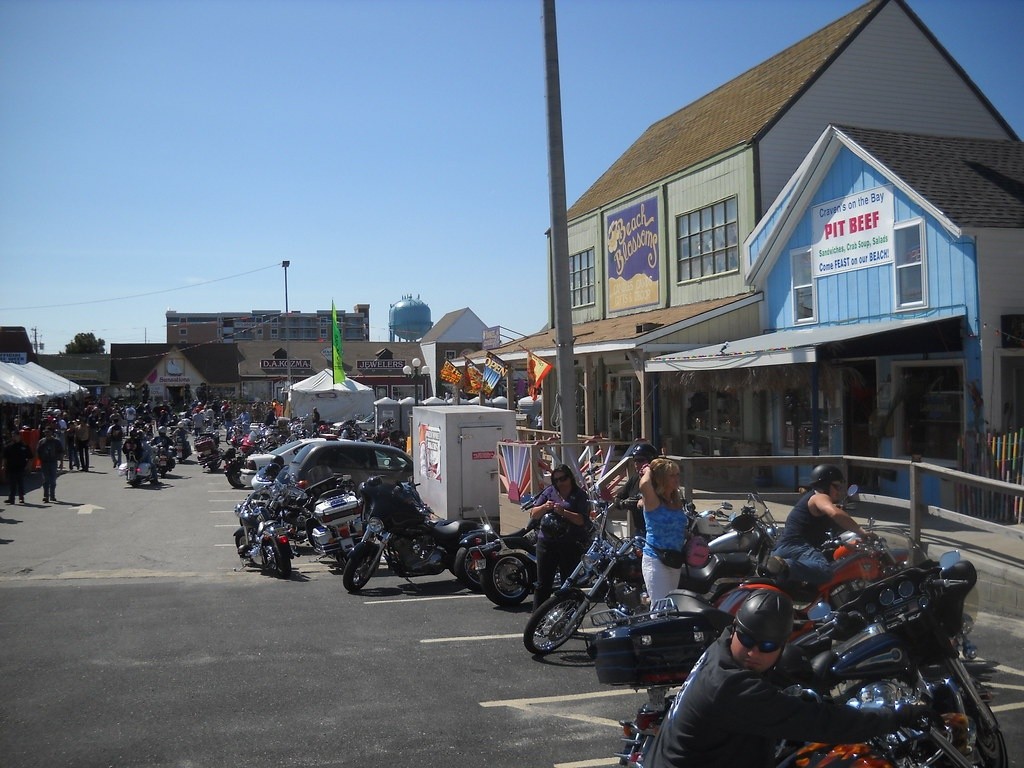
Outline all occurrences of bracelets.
[0,464,7,468]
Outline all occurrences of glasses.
[829,483,842,490]
[633,457,649,463]
[553,474,570,484]
[734,625,783,653]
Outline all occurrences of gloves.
[892,703,946,733]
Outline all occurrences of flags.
[526,351,552,400]
[440,361,464,384]
[462,358,482,394]
[480,352,510,399]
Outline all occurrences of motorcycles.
[118,410,1011,768]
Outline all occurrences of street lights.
[281,260,293,391]
[403,357,430,408]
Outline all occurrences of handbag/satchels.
[644,541,685,569]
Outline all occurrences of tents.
[282,367,378,423]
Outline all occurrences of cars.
[240,438,399,487]
[286,441,414,494]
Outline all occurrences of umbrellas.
[0,360,89,404]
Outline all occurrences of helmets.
[129,428,137,436]
[540,512,566,539]
[682,536,708,566]
[196,408,200,413]
[736,589,794,643]
[267,463,282,475]
[136,429,144,437]
[158,426,166,434]
[271,455,284,467]
[177,422,184,429]
[629,443,658,461]
[809,464,844,486]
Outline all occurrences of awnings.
[644,307,968,493]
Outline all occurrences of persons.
[35,425,64,503]
[312,405,320,424]
[756,463,876,587]
[0,396,279,484]
[251,456,285,491]
[644,588,973,768]
[611,457,687,619]
[530,465,593,639]
[1,430,34,504]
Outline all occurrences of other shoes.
[4,460,122,504]
[155,481,163,486]
[763,555,790,576]
